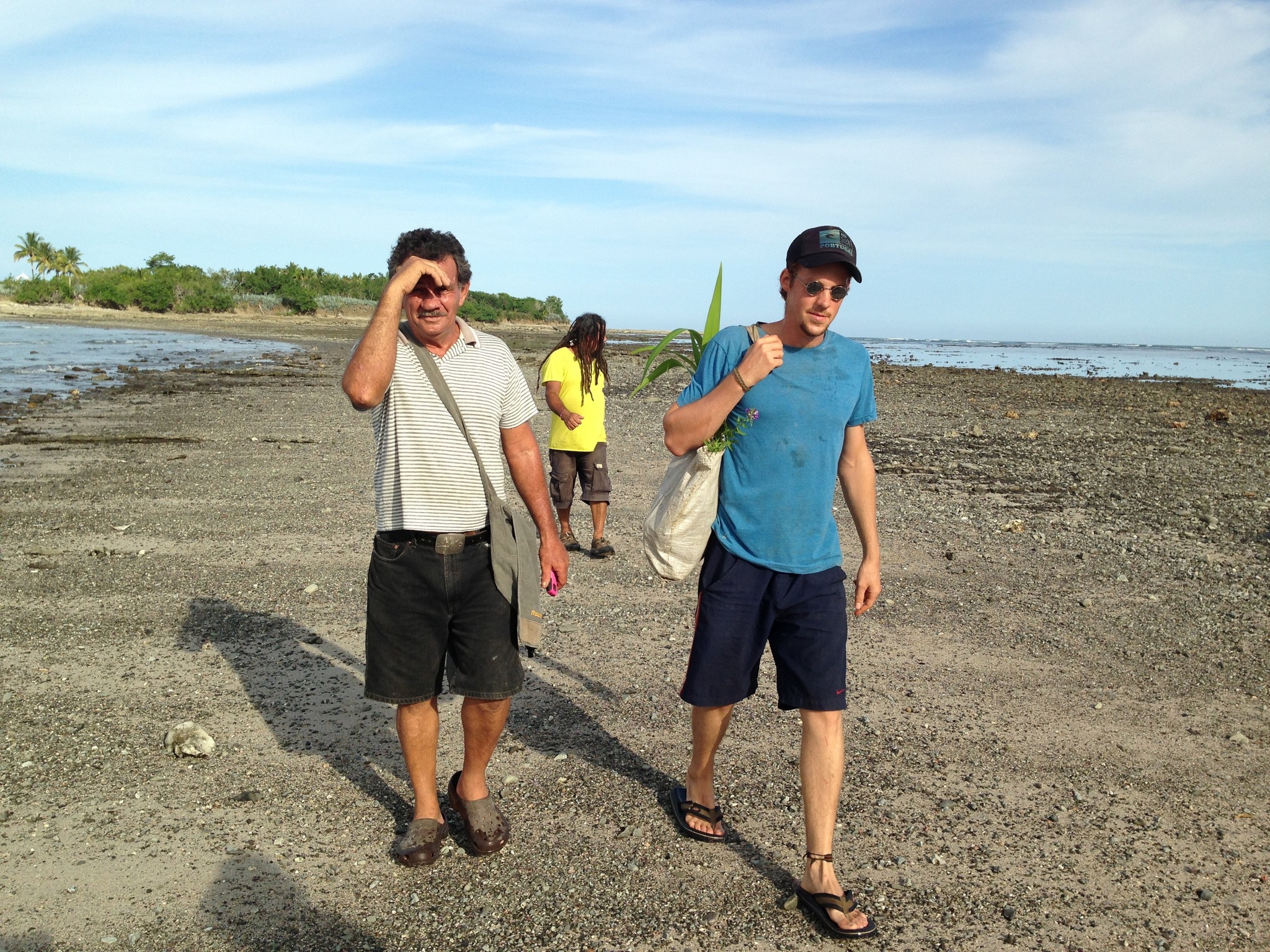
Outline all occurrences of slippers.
[796,883,878,937]
[668,786,728,844]
[448,770,509,854]
[395,811,452,867]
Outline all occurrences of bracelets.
[560,409,567,420]
[733,365,753,394]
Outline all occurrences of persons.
[537,313,615,558]
[341,228,567,866]
[662,227,882,940]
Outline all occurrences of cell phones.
[546,569,557,596]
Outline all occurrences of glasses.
[792,270,850,300]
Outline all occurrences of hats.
[787,226,862,283]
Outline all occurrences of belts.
[401,528,489,554]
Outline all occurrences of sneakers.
[560,532,580,551]
[590,537,616,555]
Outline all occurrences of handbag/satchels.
[642,325,759,581]
[488,498,543,656]
